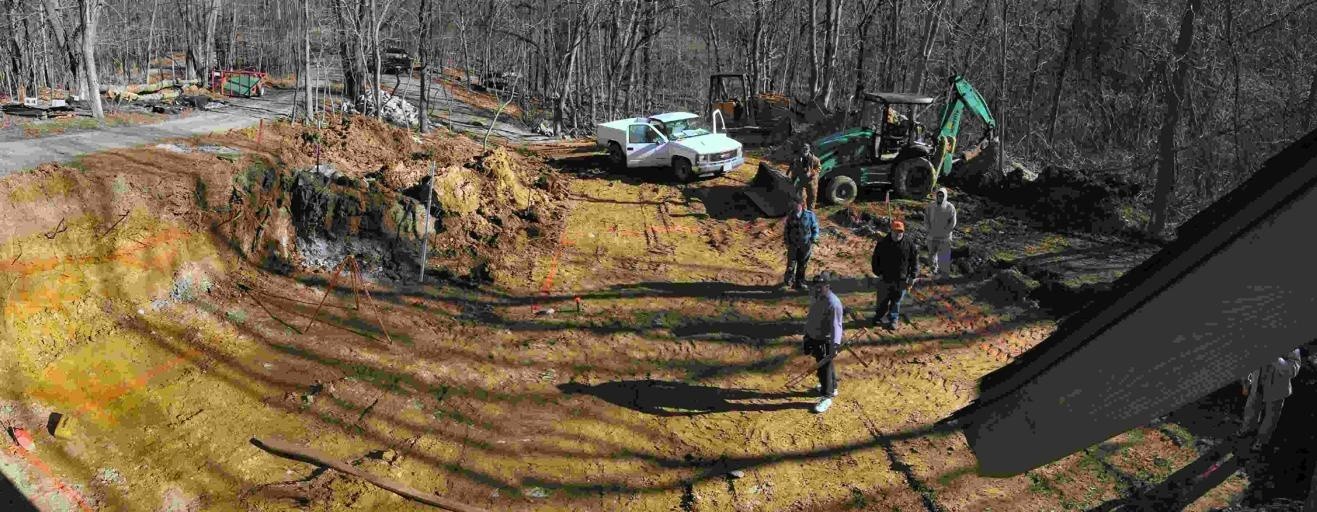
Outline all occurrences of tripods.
[302,252,395,348]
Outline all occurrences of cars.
[478,70,530,91]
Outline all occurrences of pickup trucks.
[597,109,746,181]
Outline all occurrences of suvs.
[367,44,410,75]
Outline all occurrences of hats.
[890,221,905,233]
[806,275,830,286]
[800,143,810,150]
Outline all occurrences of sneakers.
[784,276,811,292]
[1236,425,1249,438]
[888,322,898,330]
[814,398,833,413]
[817,385,839,397]
[1249,437,1264,454]
[930,265,950,284]
[872,317,883,325]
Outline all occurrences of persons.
[782,196,817,291]
[870,219,919,330]
[782,142,821,212]
[1234,349,1302,453]
[799,273,844,414]
[922,185,956,279]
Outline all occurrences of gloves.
[829,343,839,358]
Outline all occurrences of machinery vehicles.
[702,68,790,145]
[743,74,1002,218]
